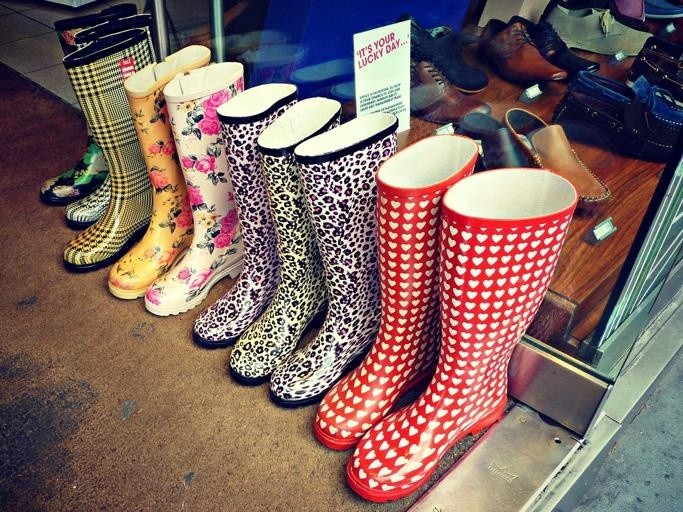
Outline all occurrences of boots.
[65,14,155,231]
[312,135,479,452]
[144,63,248,317]
[108,45,211,301]
[269,114,398,408]
[347,168,578,504]
[230,98,343,386]
[194,83,300,350]
[38,5,139,207]
[62,30,154,275]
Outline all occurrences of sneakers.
[643,37,682,63]
[402,15,491,93]
[546,6,654,58]
[552,90,680,163]
[566,70,683,130]
[629,52,683,105]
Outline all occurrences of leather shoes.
[409,59,492,127]
[453,113,535,174]
[478,19,569,86]
[503,108,612,210]
[508,16,601,79]
[645,1,683,19]
